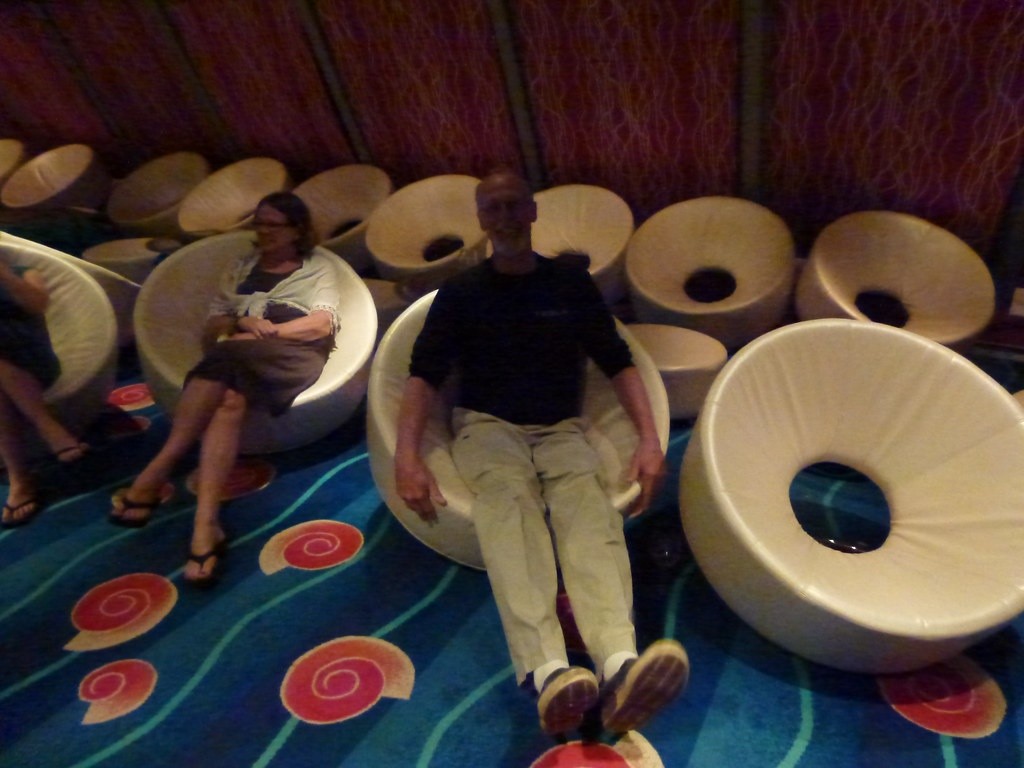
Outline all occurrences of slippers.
[109,494,162,527]
[49,441,89,466]
[184,533,227,587]
[0,496,38,528]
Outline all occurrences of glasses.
[250,219,293,232]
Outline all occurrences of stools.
[794,210,996,355]
[177,157,289,238]
[1,243,119,446]
[367,288,670,571]
[290,165,395,273]
[366,174,494,288]
[106,151,209,230]
[630,196,794,348]
[0,144,94,208]
[0,231,142,347]
[486,184,634,306]
[132,231,378,453]
[678,319,1024,671]
[82,238,180,286]
[621,320,726,419]
[0,140,23,179]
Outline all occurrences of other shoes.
[537,639,690,734]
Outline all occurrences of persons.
[108,192,341,590]
[394,167,688,744]
[1,262,97,527]
